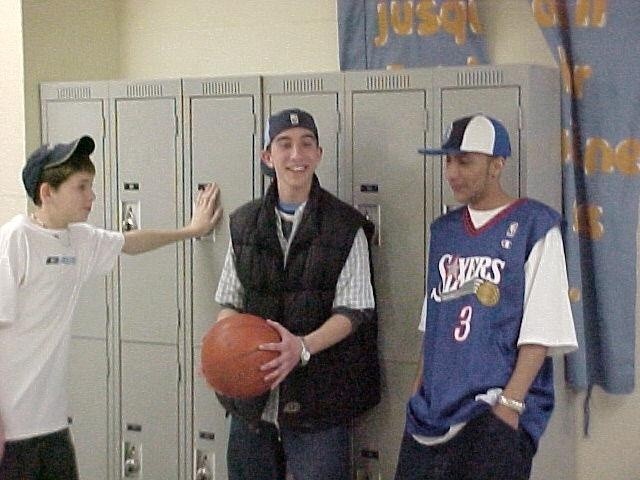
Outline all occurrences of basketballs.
[200,314,282,397]
[477,283,503,306]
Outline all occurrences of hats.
[21,135,96,197]
[258,108,319,176]
[415,114,512,159]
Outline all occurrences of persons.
[1,131,225,479]
[195,105,386,480]
[392,111,582,480]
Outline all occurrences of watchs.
[298,336,312,370]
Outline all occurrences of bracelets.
[497,393,529,417]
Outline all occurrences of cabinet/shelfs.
[349,364,422,477]
[115,342,185,477]
[262,72,344,203]
[529,352,564,476]
[109,77,183,341]
[434,64,561,217]
[345,68,435,363]
[40,80,112,340]
[187,74,263,345]
[185,346,232,475]
[65,340,114,476]
